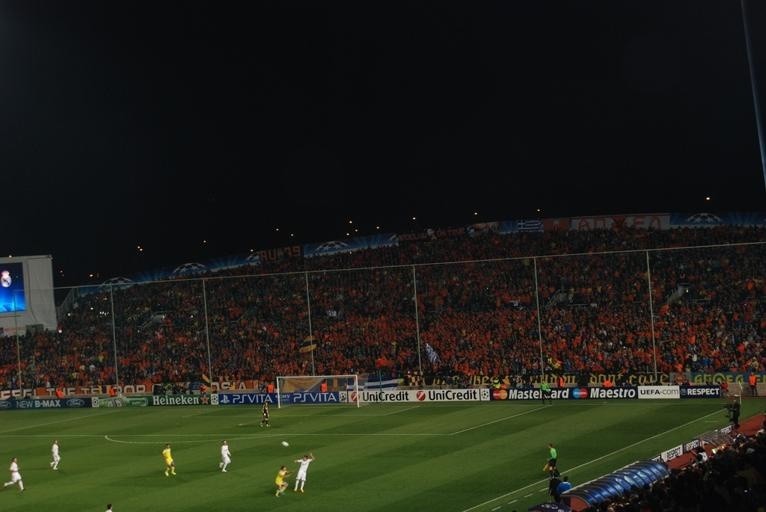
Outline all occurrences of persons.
[293,453,315,493]
[724,403,733,417]
[275,465,293,497]
[730,399,742,430]
[0,224,766,398]
[161,443,176,477]
[540,379,552,405]
[219,440,232,473]
[49,439,61,471]
[105,504,113,512]
[3,457,26,491]
[258,402,270,428]
[546,419,766,512]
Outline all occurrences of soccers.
[282,441,289,447]
